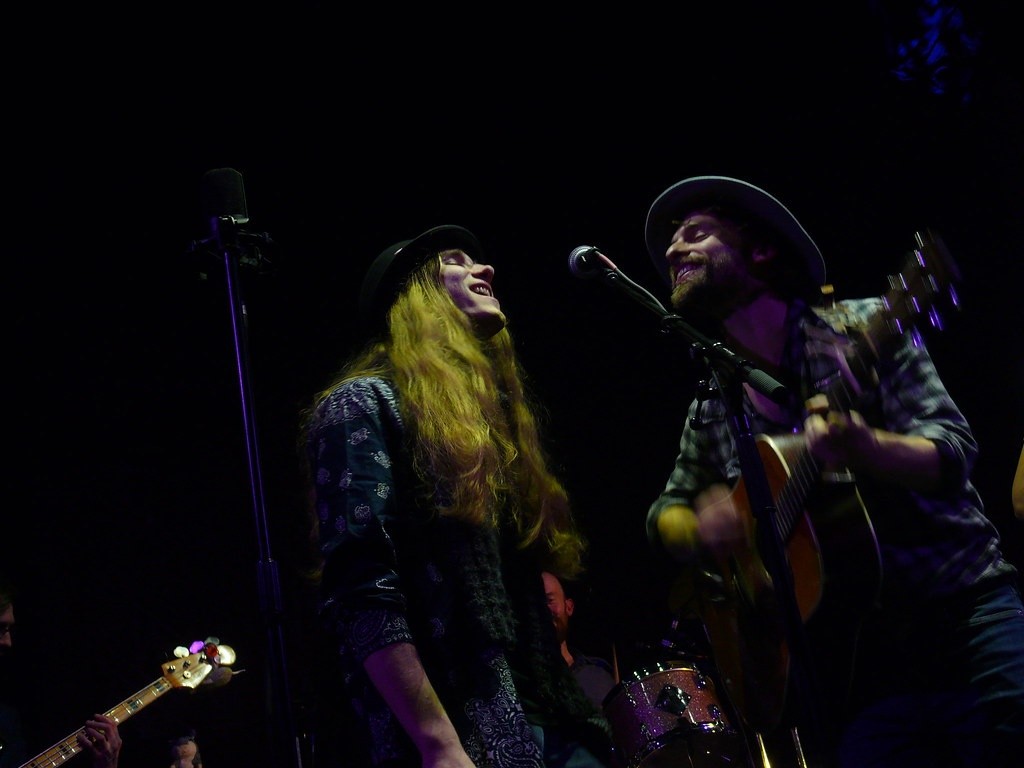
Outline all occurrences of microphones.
[204,168,250,255]
[568,245,602,278]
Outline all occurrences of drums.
[602,658,735,767]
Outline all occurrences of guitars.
[696,228,963,732]
[15,635,238,768]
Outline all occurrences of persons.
[0,593,122,768]
[301,225,588,768]
[171,741,202,768]
[646,177,1024,768]
[541,573,615,674]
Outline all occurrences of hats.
[642,175,826,305]
[357,225,487,341]
